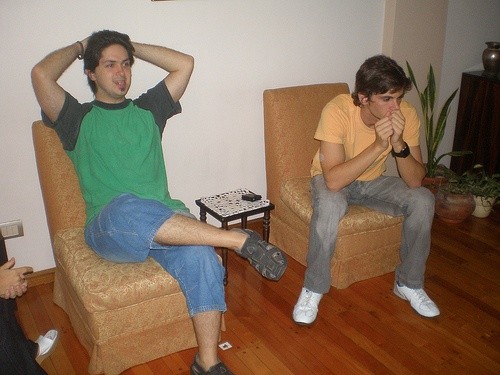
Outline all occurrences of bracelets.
[76,41,84,60]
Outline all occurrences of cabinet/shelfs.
[449,69,499,175]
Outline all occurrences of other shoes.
[35,329,60,366]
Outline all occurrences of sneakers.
[231,227,288,282]
[393,282,441,319]
[292,287,323,326]
[190,352,234,375]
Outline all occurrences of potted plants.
[465,167,500,218]
[406,59,473,197]
[435,169,475,224]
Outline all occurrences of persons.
[293,54,441,324]
[31,28,288,375]
[0,232,60,375]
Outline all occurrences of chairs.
[262,82,406,290]
[31,120,226,375]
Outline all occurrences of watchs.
[391,141,410,158]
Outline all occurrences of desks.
[195,188,275,286]
